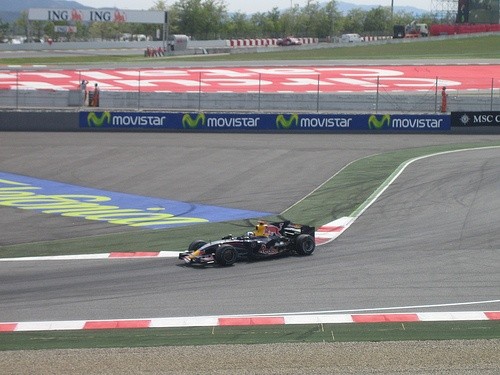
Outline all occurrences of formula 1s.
[177,220,316,267]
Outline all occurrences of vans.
[404,23,429,38]
[342,33,364,43]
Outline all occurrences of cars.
[275,37,302,46]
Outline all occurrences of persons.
[78,79,89,105]
[442,86,448,112]
[93,83,99,107]
[147,46,165,57]
